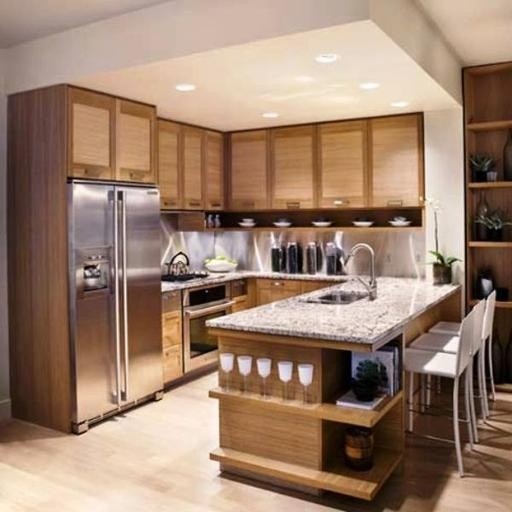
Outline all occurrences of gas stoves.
[159,270,225,283]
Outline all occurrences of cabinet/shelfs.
[367,111,422,207]
[205,128,226,211]
[229,280,252,313]
[157,115,182,208]
[161,288,184,394]
[205,325,400,500]
[255,278,346,307]
[459,60,512,394]
[270,124,321,211]
[320,117,370,210]
[224,127,272,212]
[178,120,207,212]
[8,82,159,188]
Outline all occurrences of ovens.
[181,282,236,374]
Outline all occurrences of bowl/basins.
[206,262,238,273]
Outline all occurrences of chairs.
[403,290,497,479]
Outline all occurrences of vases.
[341,424,376,472]
[430,261,452,285]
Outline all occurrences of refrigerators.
[65,177,164,435]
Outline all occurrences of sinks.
[298,289,370,306]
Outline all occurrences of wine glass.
[236,356,255,396]
[217,351,237,393]
[277,360,293,405]
[297,364,315,408]
[256,357,272,401]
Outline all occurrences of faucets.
[342,243,376,283]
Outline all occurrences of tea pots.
[165,251,190,275]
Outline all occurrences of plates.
[352,221,375,226]
[310,221,333,226]
[388,220,412,226]
[238,223,257,228]
[273,222,291,227]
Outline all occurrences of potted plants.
[466,149,500,182]
[471,206,511,242]
[347,356,391,402]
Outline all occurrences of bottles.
[491,206,505,242]
[475,265,495,299]
[208,214,221,227]
[502,128,511,181]
[271,242,282,271]
[477,189,490,239]
[325,242,338,276]
[286,240,298,274]
[345,432,375,472]
[304,242,317,274]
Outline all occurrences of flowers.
[416,192,464,269]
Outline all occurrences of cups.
[487,171,497,181]
[496,286,509,301]
[277,216,287,222]
[393,216,407,221]
[242,218,254,222]
